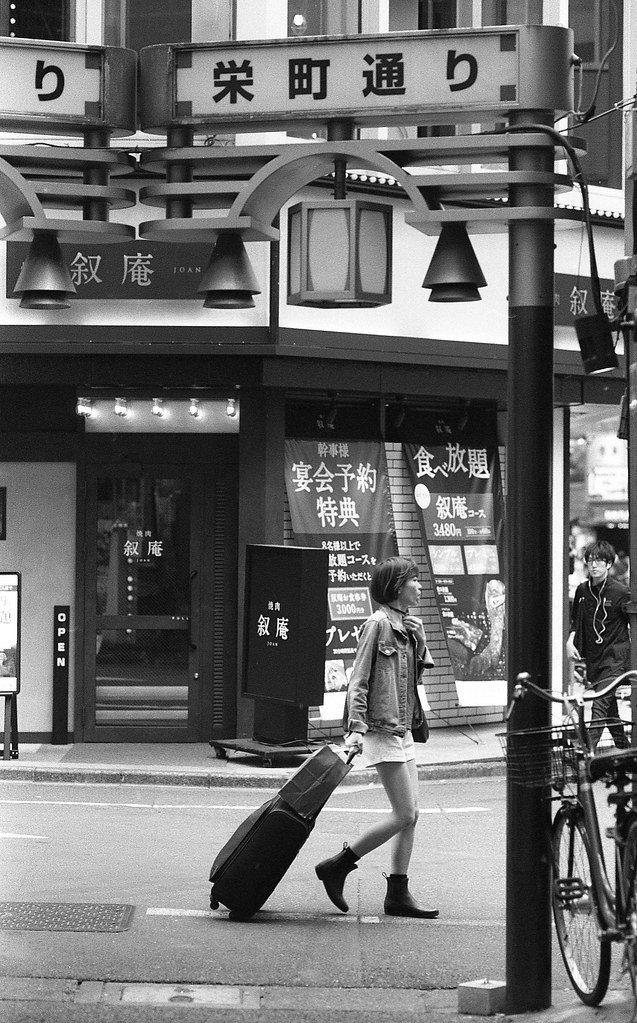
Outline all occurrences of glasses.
[585,557,608,563]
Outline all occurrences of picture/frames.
[0,570,22,693]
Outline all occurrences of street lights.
[284,108,556,1013]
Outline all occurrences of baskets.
[495,718,635,788]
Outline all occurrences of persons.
[315,557,439,916]
[566,541,631,749]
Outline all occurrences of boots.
[382,872,439,919]
[315,842,359,912]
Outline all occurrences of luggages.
[209,744,360,921]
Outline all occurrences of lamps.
[286,119,393,311]
[226,398,236,416]
[188,398,201,417]
[114,398,128,416]
[421,219,489,304]
[11,230,77,310]
[76,397,93,416]
[196,234,260,309]
[152,398,165,417]
[574,313,620,377]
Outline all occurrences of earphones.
[606,563,609,569]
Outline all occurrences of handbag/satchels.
[577,583,584,658]
[412,710,429,743]
[277,745,353,819]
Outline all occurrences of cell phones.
[605,601,613,606]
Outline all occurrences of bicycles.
[494,671,637,1009]
